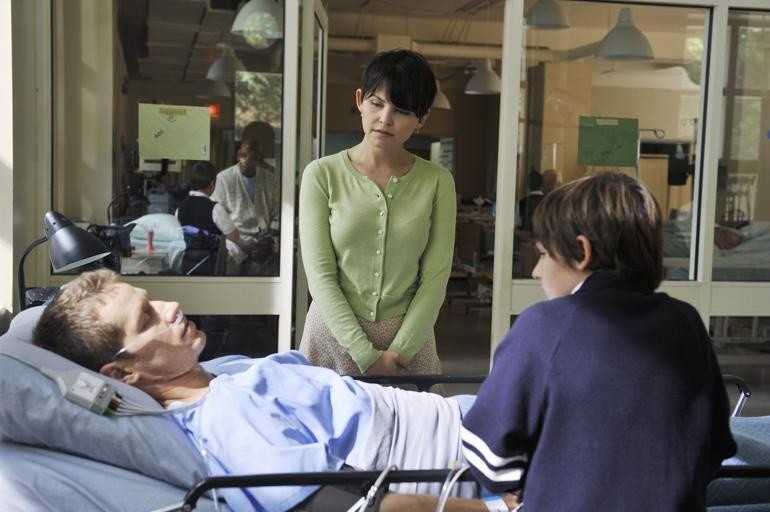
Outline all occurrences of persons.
[714,208,747,249]
[175,160,253,255]
[542,169,563,191]
[515,171,545,230]
[212,138,280,275]
[34,268,519,511]
[297,47,458,396]
[460,172,738,511]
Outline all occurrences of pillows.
[124,212,182,241]
[0,306,223,500]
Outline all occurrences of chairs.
[169,226,228,275]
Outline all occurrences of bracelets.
[481,496,510,512]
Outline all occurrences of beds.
[108,192,180,250]
[662,221,770,281]
[0,308,770,512]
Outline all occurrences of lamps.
[18,210,112,311]
[230,0,283,50]
[595,8,655,61]
[464,58,501,95]
[431,64,452,110]
[527,0,570,30]
[206,49,248,97]
